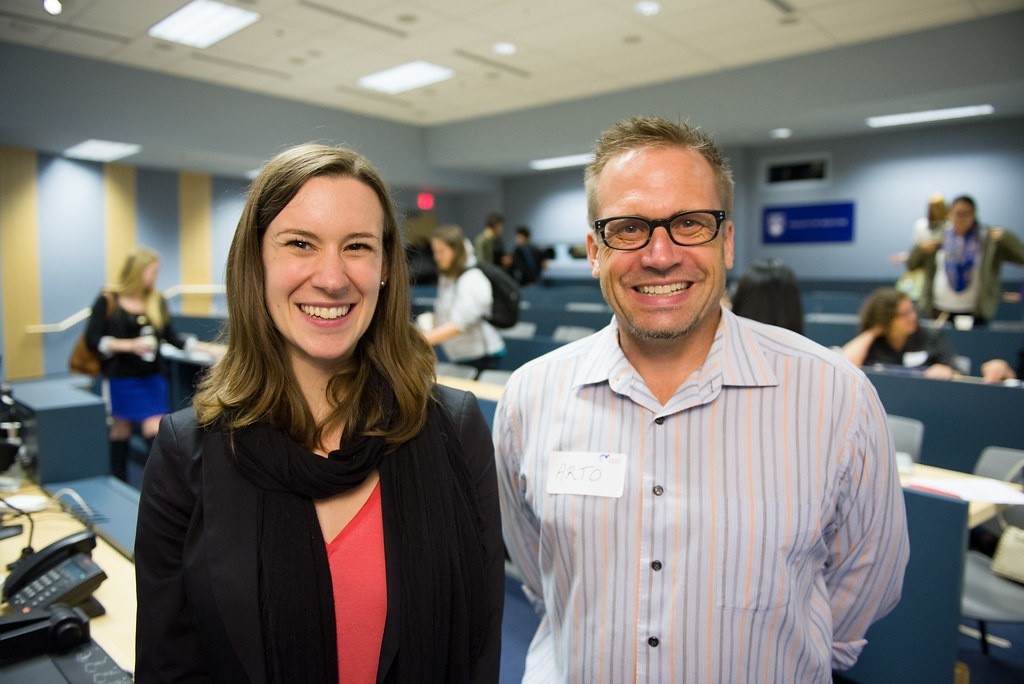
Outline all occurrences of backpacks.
[456,263,520,329]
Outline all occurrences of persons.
[87,248,224,484]
[475,213,506,266]
[731,263,803,334]
[510,227,547,287]
[908,197,1024,324]
[841,286,961,380]
[982,343,1024,384]
[491,118,910,684]
[133,142,506,684]
[424,223,505,370]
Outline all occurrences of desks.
[0,279,1024,684]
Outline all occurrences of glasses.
[595,210,726,250]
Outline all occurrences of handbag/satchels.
[71,292,115,376]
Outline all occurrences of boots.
[109,439,128,484]
[146,435,154,449]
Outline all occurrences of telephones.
[2,529,108,618]
